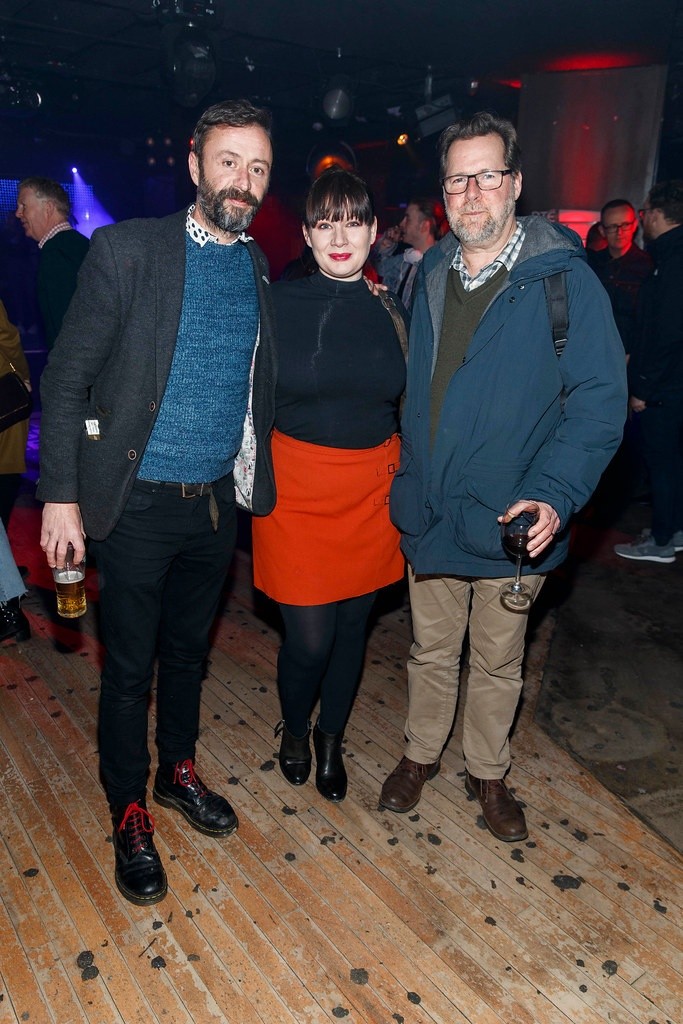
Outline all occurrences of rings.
[551,533,554,536]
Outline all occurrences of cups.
[52,542,87,618]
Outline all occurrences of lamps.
[317,75,359,132]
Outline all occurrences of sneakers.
[613,534,676,563]
[642,525,683,552]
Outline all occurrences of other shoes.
[16,565,30,580]
[0,596,32,648]
[624,464,650,512]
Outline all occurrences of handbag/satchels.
[0,368,33,435]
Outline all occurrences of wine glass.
[499,499,540,601]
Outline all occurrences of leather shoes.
[464,769,528,841]
[274,717,313,785]
[311,714,347,802]
[379,756,443,812]
[110,798,167,906]
[153,756,239,837]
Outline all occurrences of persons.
[0,179,89,641]
[38,102,387,904]
[372,194,444,317]
[379,111,627,843]
[577,177,682,564]
[252,167,412,802]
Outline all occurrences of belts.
[135,471,235,531]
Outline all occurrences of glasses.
[602,217,638,233]
[440,166,517,195]
[638,207,656,219]
